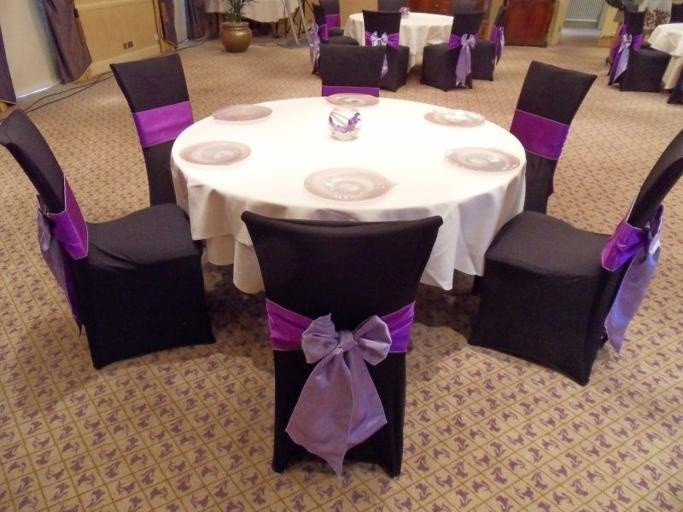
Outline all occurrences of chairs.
[111,47,195,205]
[0,104,218,368]
[605,0,681,106]
[239,205,444,477]
[465,123,682,386]
[502,58,595,215]
[305,0,511,99]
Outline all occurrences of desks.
[165,85,528,291]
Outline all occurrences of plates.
[447,147,523,173]
[212,103,273,120]
[303,167,393,201]
[427,109,485,128]
[327,93,379,108]
[179,143,250,165]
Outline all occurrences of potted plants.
[219,0,256,53]
